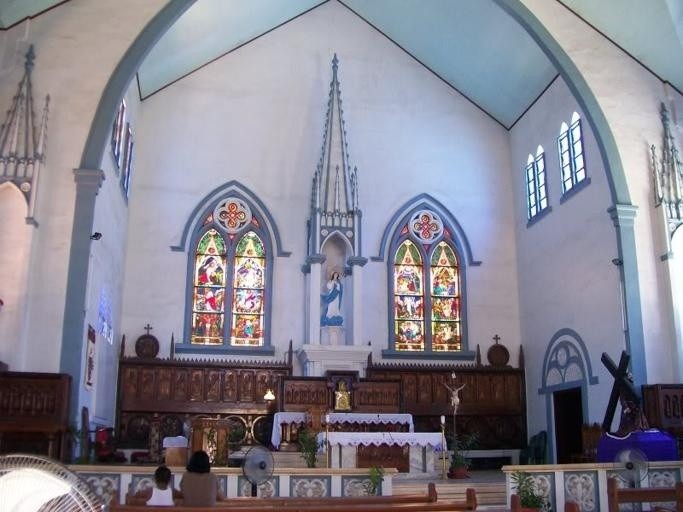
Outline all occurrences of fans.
[611,443,650,511]
[0,447,104,511]
[241,442,277,496]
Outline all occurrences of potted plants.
[448,427,485,478]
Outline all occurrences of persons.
[325,272,341,319]
[136,466,183,507]
[179,450,225,506]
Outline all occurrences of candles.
[440,414,445,425]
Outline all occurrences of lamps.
[262,387,277,403]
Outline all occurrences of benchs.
[452,444,523,467]
[508,478,682,510]
[108,482,478,511]
